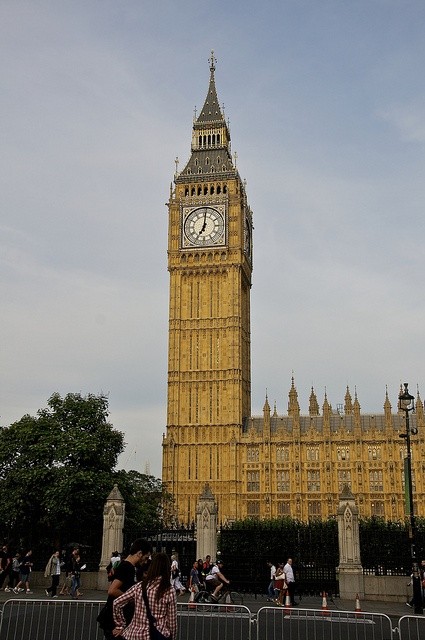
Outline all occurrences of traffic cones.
[284,589,292,615]
[354,594,363,619]
[319,591,331,615]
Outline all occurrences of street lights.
[398,383,423,613]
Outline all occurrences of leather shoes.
[292,602,299,606]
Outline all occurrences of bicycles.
[193,580,243,613]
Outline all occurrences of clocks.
[179,199,230,252]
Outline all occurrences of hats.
[216,559,224,564]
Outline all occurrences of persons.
[111,551,121,566]
[198,559,203,568]
[4,553,23,591]
[0,546,13,588]
[113,552,178,640]
[275,564,285,606]
[97,538,153,640]
[44,551,65,597]
[205,560,230,600]
[267,561,279,601]
[69,555,81,596]
[170,555,186,596]
[60,547,77,595]
[12,548,33,595]
[406,561,425,608]
[189,562,204,590]
[283,559,299,606]
[203,555,214,572]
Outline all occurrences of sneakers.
[211,594,218,601]
[13,589,19,594]
[45,589,49,596]
[5,588,11,592]
[52,595,58,599]
[77,592,83,596]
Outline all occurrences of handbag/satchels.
[141,581,174,640]
[66,578,73,587]
[282,580,288,589]
